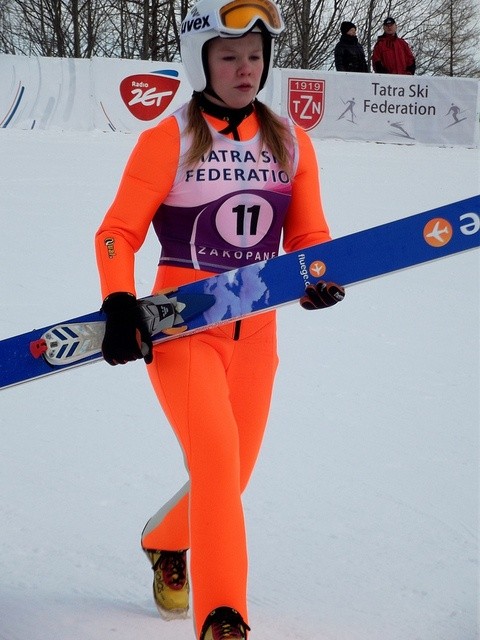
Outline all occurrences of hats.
[341,21,356,35]
[384,17,395,26]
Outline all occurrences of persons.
[372,17,417,75]
[334,21,369,73]
[93,0,346,640]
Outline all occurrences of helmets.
[180,0,275,96]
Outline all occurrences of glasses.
[180,0,286,39]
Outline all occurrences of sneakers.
[140,541,189,620]
[199,605,248,640]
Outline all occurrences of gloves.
[101,291,153,366]
[299,281,344,310]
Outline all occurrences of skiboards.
[0,194,480,391]
